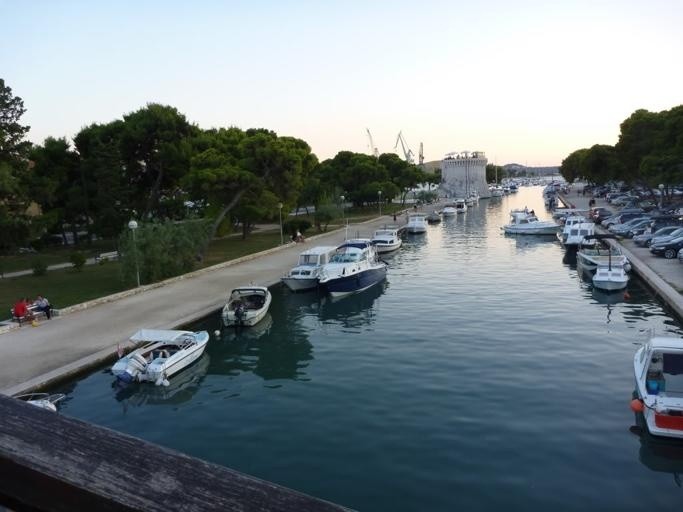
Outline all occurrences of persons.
[34,294,52,319]
[292,231,298,241]
[14,297,32,323]
[588,197,595,206]
[296,229,303,241]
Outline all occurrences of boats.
[220,280,274,324]
[109,326,210,382]
[551,204,631,293]
[223,313,274,343]
[630,327,683,447]
[114,351,209,409]
[13,389,68,416]
[280,173,569,299]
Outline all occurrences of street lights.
[127,220,145,292]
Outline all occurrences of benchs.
[9,303,53,327]
[96,250,117,263]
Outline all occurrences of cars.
[579,181,683,267]
